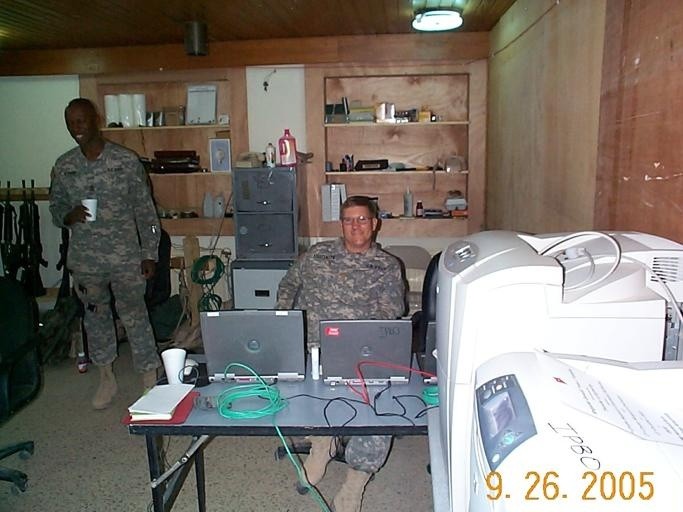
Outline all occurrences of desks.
[126,352,435,512]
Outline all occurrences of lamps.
[411,7,464,31]
[186,20,208,55]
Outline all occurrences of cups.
[81,199,97,222]
[160,348,187,383]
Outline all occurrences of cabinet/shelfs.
[316,58,490,219]
[78,67,236,235]
[232,167,310,309]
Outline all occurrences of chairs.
[276,245,431,477]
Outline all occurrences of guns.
[56,228,69,296]
[0,180,5,277]
[30,179,47,295]
[4,181,16,279]
[18,179,34,296]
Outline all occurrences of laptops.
[200,310,308,382]
[415,319,439,386]
[317,320,412,388]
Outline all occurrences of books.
[130,386,173,422]
[120,392,200,425]
[128,384,196,413]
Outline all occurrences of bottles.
[77,352,89,374]
[416,200,423,216]
[265,144,276,168]
[403,187,412,216]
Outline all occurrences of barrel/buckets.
[279,129,298,167]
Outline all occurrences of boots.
[295,435,339,494]
[330,468,372,512]
[92,365,118,410]
[143,368,157,391]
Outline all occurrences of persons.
[49,98,162,409]
[275,195,408,512]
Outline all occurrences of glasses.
[342,215,370,226]
